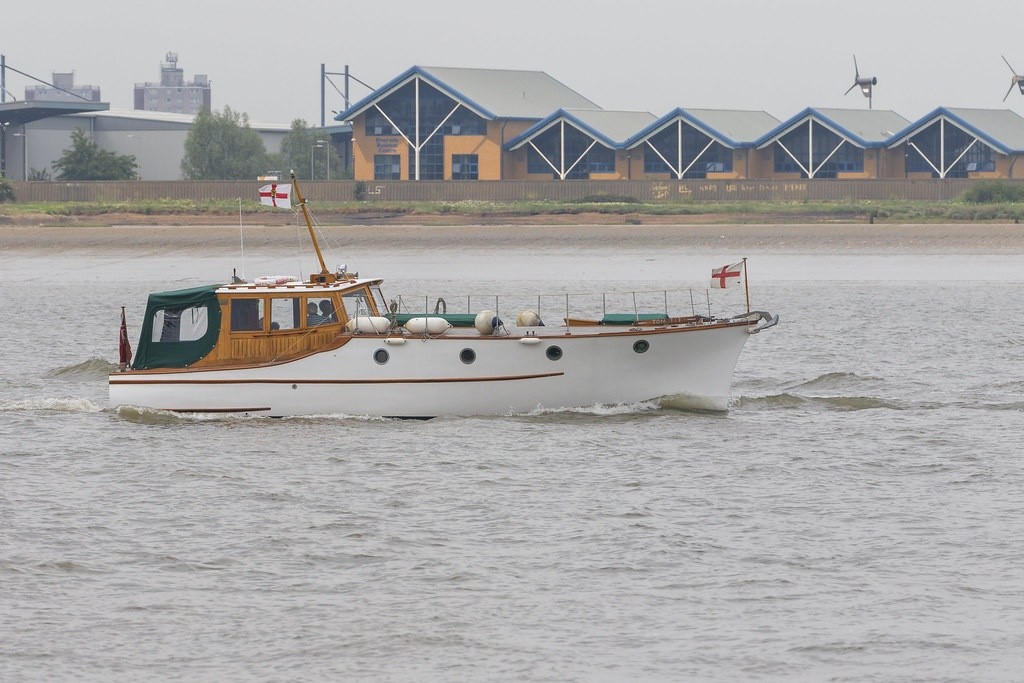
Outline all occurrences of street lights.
[311,144,323,180]
[317,140,329,181]
[129,134,139,179]
[14,133,28,181]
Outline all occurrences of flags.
[119,319,132,372]
[258,183,292,209]
[710,262,743,289]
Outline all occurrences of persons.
[307,300,334,325]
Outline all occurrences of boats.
[108,170,779,420]
